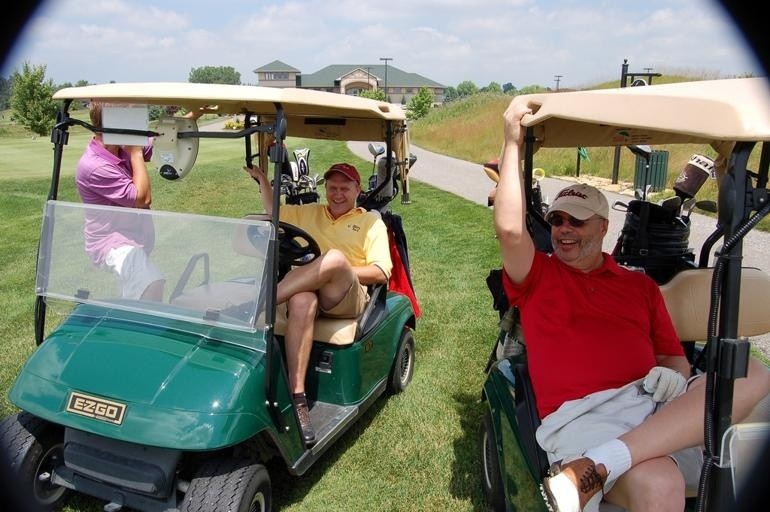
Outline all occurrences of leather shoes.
[537,447,611,512]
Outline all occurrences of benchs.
[518,265,770,500]
[169,212,386,347]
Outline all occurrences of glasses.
[545,212,605,228]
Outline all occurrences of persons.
[493,92,770,512]
[74,98,209,303]
[202,163,394,445]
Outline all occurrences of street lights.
[365,67,375,91]
[608,55,667,189]
[380,56,394,102]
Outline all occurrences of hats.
[543,181,611,224]
[323,161,361,186]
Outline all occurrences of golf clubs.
[612,184,718,221]
[277,144,325,195]
[366,143,417,201]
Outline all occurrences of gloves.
[641,364,688,404]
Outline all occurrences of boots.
[288,390,318,445]
